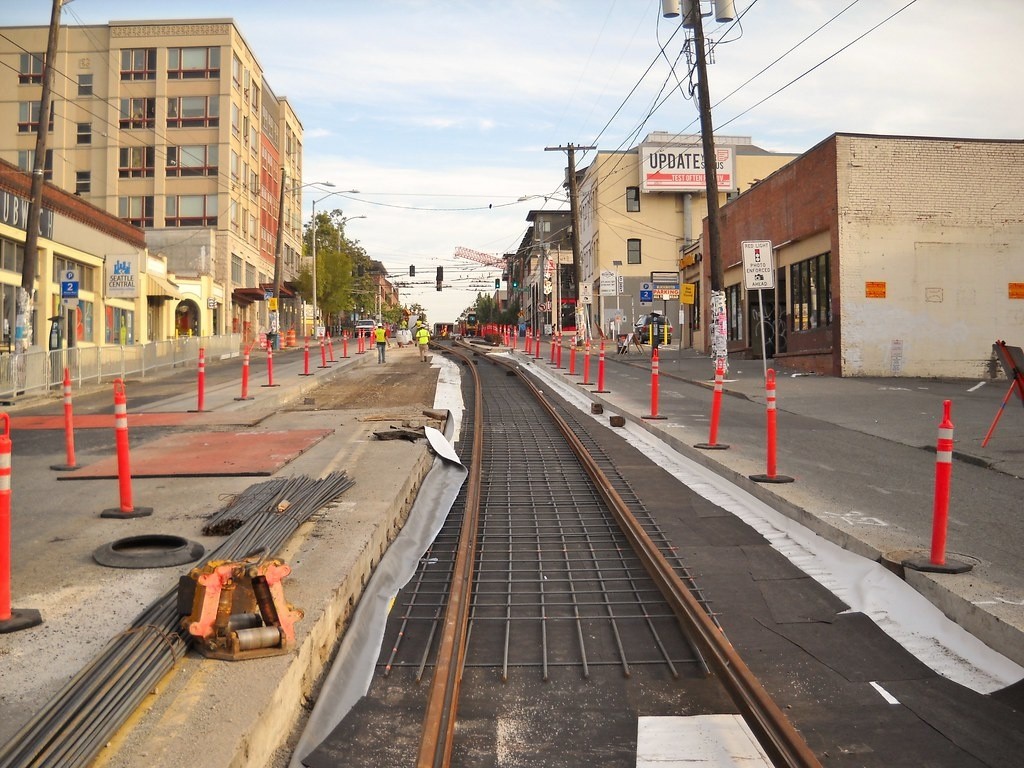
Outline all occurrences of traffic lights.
[495,279,500,288]
[513,277,518,287]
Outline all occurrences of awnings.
[146,274,184,301]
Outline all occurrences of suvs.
[630,313,673,345]
[354,319,378,338]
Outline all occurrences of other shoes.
[425,356,427,361]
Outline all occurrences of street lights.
[518,193,586,347]
[313,189,361,339]
[337,215,369,252]
[272,171,335,313]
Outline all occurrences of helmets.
[378,322,383,326]
[420,324,424,327]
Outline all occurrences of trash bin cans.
[616,333,628,354]
[265,332,279,351]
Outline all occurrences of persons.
[374,323,390,365]
[310,326,315,336]
[442,330,446,340]
[415,324,430,362]
[411,318,425,331]
[400,319,408,330]
